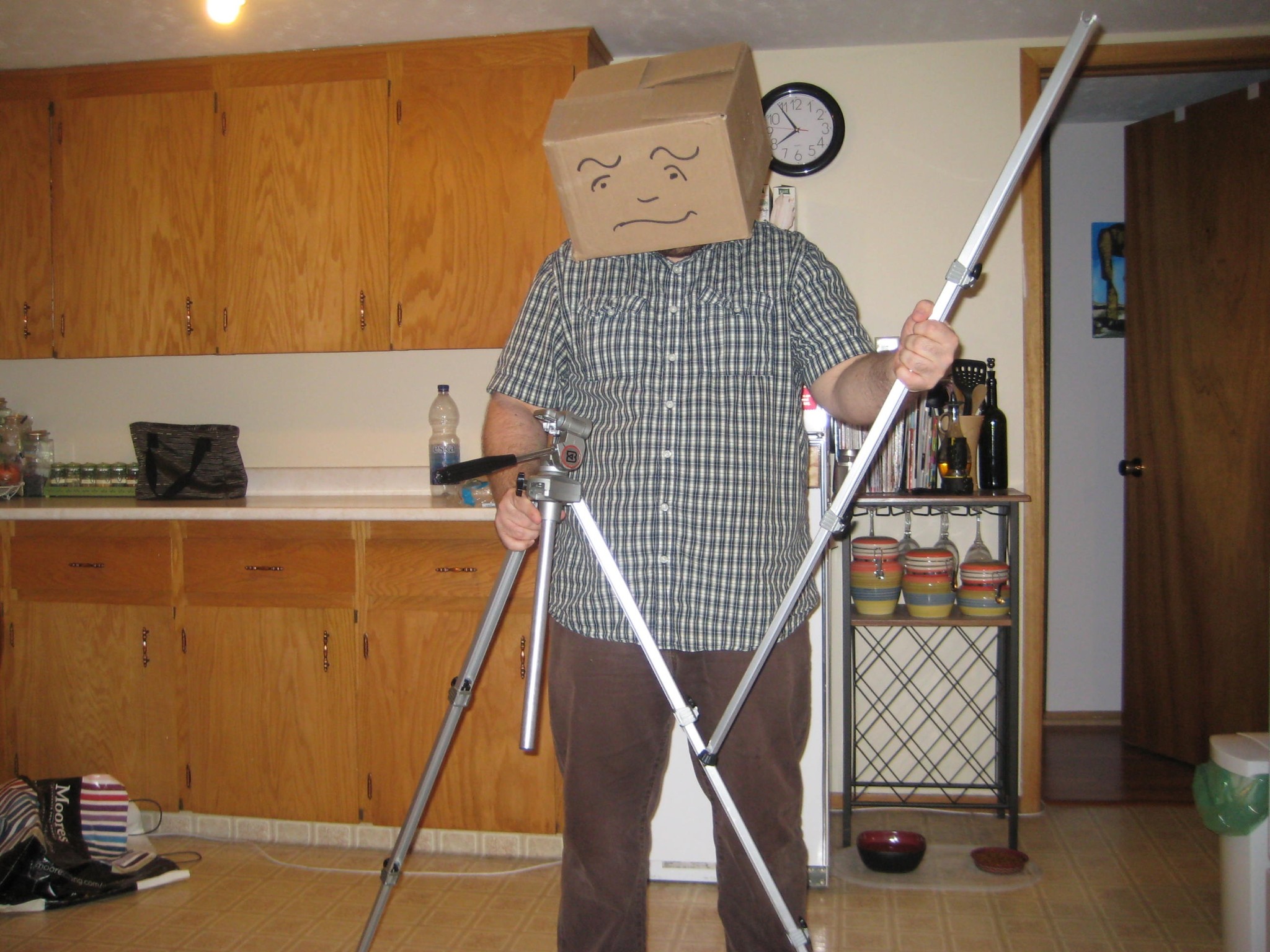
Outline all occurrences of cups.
[934,415,985,491]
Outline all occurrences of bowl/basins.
[969,847,1029,874]
[856,829,926,873]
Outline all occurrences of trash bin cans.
[1209,733,1270,952]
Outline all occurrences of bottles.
[0,398,54,477]
[49,461,139,486]
[428,384,461,498]
[954,560,1011,618]
[850,536,903,614]
[900,548,956,619]
[935,391,973,495]
[979,370,1008,490]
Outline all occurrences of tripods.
[356,409,816,952]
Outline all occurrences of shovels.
[953,358,988,416]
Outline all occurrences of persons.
[481,41,958,952]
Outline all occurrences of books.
[829,389,939,494]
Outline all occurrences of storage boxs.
[542,42,771,262]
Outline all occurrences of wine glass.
[931,505,959,574]
[858,505,887,537]
[893,505,923,565]
[964,505,993,561]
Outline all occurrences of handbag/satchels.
[129,422,248,500]
[0,774,201,911]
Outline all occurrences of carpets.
[829,844,1045,892]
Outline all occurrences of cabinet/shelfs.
[0,27,613,360]
[0,519,565,834]
[842,488,1031,850]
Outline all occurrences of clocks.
[761,82,845,177]
